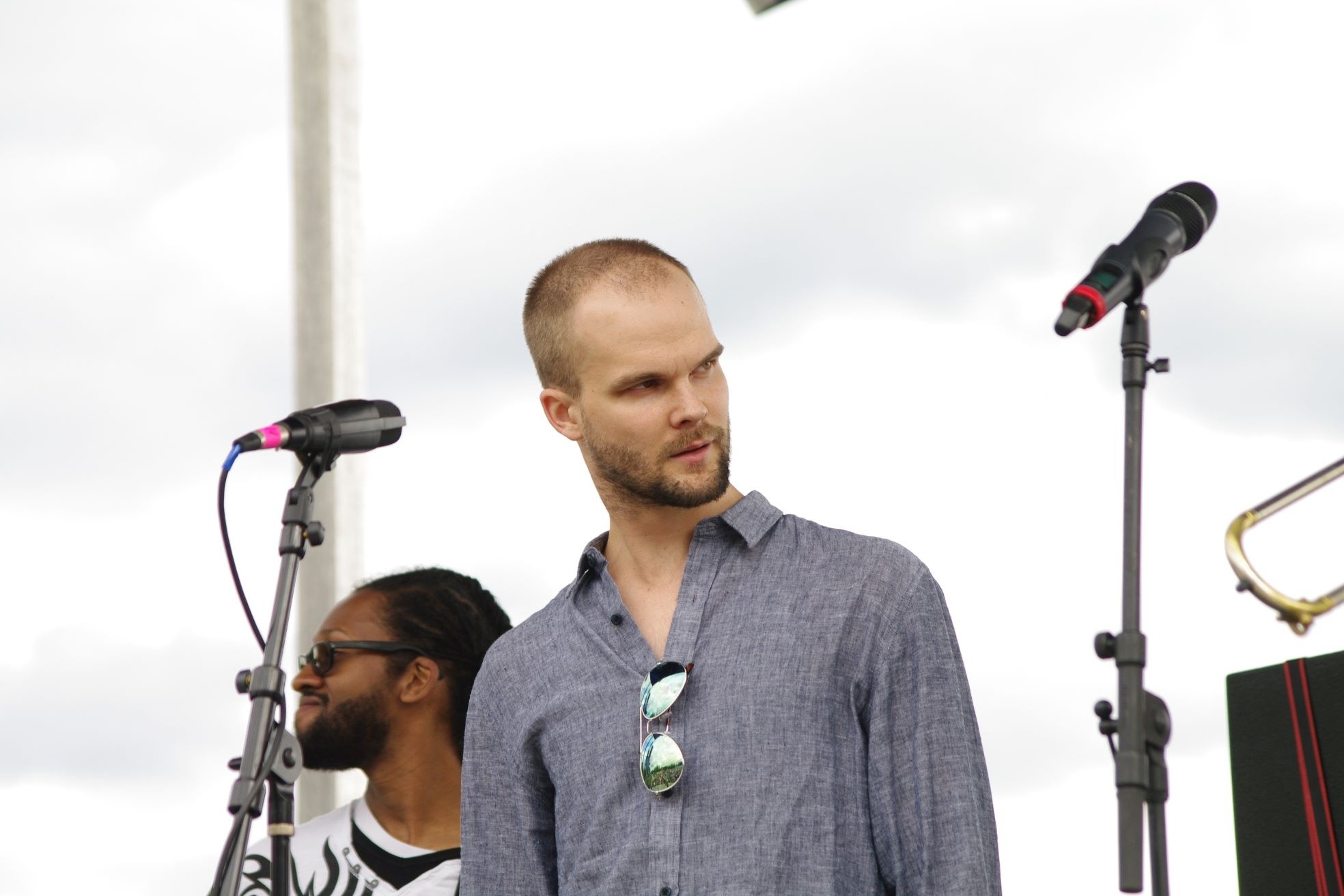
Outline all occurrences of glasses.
[297,641,446,681]
[640,660,697,797]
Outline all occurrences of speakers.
[1225,652,1344,895]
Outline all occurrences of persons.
[460,238,1006,896]
[238,566,513,896]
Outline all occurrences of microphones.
[232,399,402,456]
[1054,181,1218,337]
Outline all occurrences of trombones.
[1224,455,1344,637]
